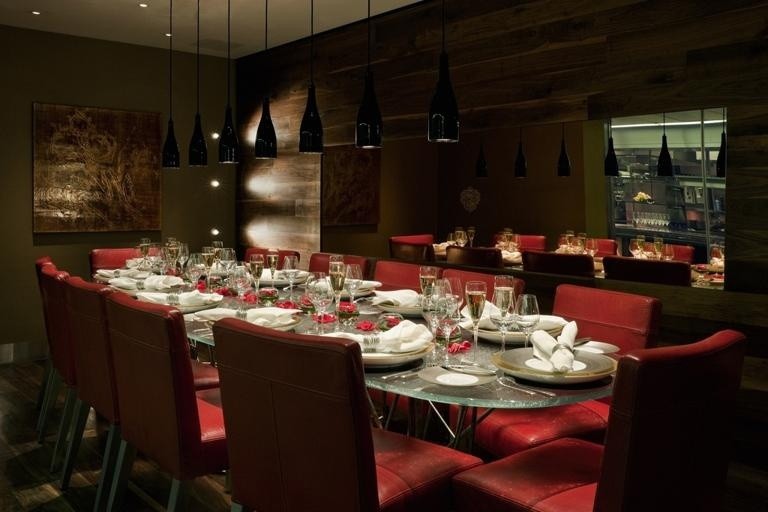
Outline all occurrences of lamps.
[355,2,383,150]
[298,1,324,154]
[219,0,240,165]
[188,0,208,166]
[428,0,461,143]
[162,1,182,169]
[253,1,277,160]
[470,101,731,183]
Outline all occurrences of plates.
[693,260,724,283]
[94,258,137,295]
[495,339,622,387]
[377,291,424,319]
[297,325,434,369]
[472,312,568,345]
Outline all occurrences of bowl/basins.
[713,223,725,235]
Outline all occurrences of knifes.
[381,368,426,383]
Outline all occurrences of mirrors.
[316,104,728,293]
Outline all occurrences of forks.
[493,376,557,398]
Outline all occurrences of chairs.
[105,292,228,512]
[244,247,300,269]
[33,256,108,489]
[453,329,747,512]
[88,248,162,276]
[447,284,663,459]
[212,317,484,511]
[366,268,524,423]
[374,261,442,296]
[309,253,372,283]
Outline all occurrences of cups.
[714,196,725,211]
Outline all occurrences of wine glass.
[629,233,676,262]
[631,211,672,232]
[563,230,600,263]
[138,237,376,325]
[444,226,523,260]
[419,266,540,373]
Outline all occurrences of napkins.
[359,279,382,290]
[196,307,304,327]
[136,288,223,306]
[109,274,182,290]
[370,289,419,306]
[324,319,432,353]
[458,300,567,331]
[530,321,577,373]
